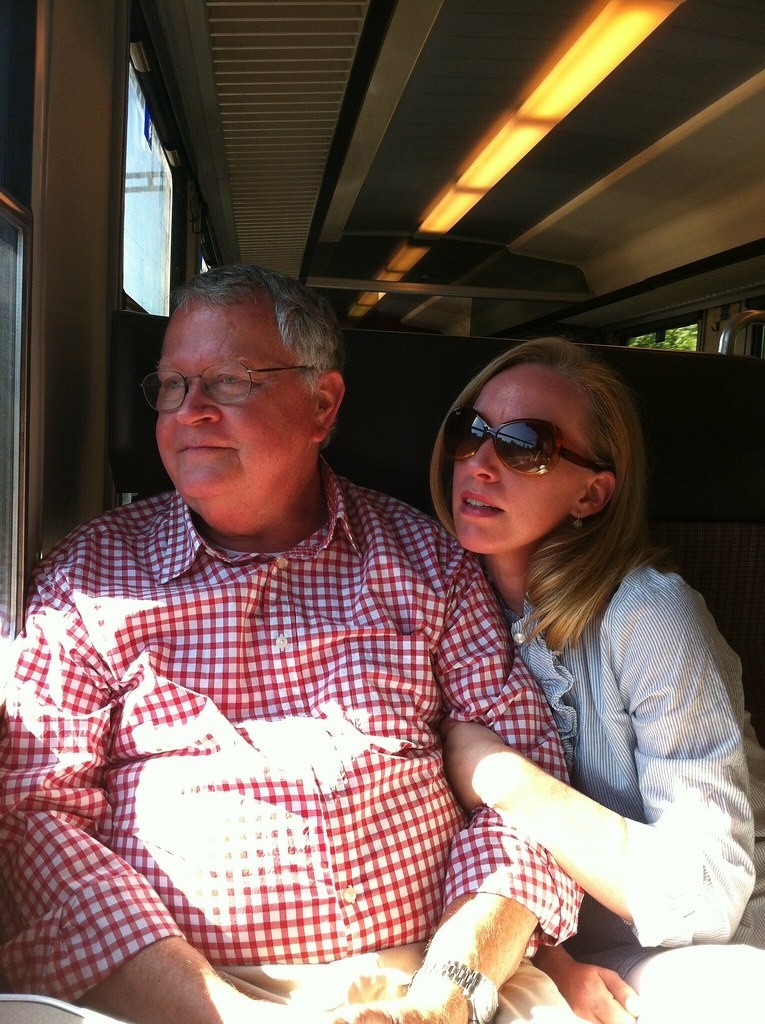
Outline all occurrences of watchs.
[423,948,500,1023]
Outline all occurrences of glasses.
[443,405,608,476]
[141,361,312,410]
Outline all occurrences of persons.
[0,259,588,1024]
[429,334,765,1023]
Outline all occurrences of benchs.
[109,312,765,751]
